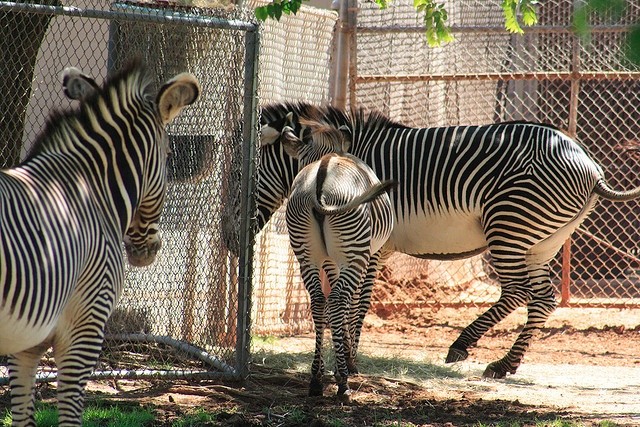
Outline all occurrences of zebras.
[220,96,640,379]
[0,57,199,427]
[282,120,400,399]
